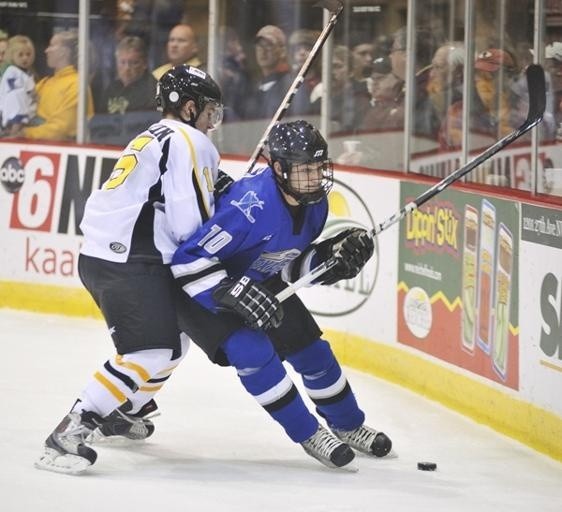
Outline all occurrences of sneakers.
[93,413,154,441]
[299,426,355,469]
[46,412,97,466]
[329,423,392,458]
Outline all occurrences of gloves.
[217,274,284,330]
[333,229,374,279]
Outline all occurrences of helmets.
[268,120,334,205]
[155,65,223,128]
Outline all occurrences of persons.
[44,63,236,464]
[0,0,562,147]
[167,120,393,470]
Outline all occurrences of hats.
[255,25,286,50]
[361,55,392,77]
[474,47,513,73]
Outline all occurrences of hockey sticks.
[275,65,545,302]
[244,0,345,177]
